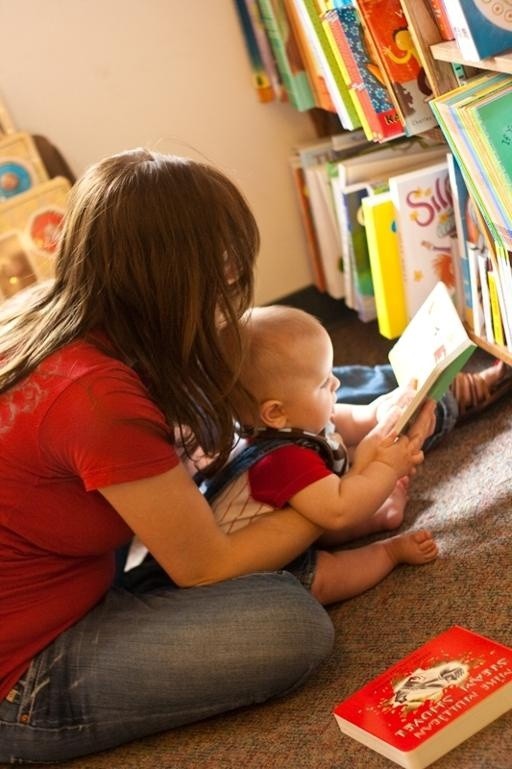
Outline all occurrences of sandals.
[449,361,512,421]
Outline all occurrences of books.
[236,0,511,352]
[333,624,512,769]
[386,281,476,441]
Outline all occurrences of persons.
[137,305,438,608]
[0,146,510,766]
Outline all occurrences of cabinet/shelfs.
[303,0,509,371]
